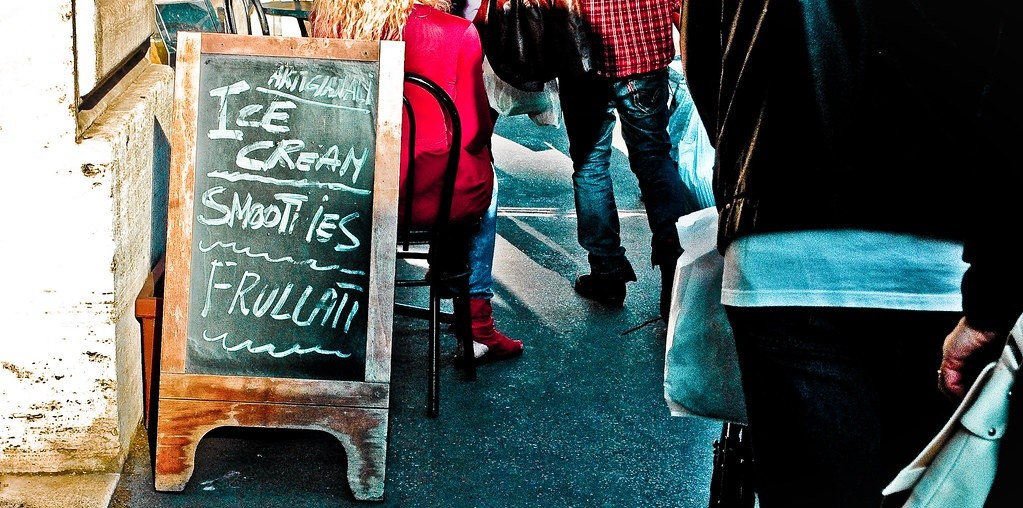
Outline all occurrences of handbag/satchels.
[659,206,752,429]
[882,341,1017,508]
[469,0,567,130]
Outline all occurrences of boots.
[573,253,637,312]
[468,297,522,359]
[645,233,685,327]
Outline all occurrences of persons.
[310,0,522,356]
[551,0,689,308]
[676,0,1023,508]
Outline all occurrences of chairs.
[392,70,490,420]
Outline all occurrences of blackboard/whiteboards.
[152,30,407,503]
